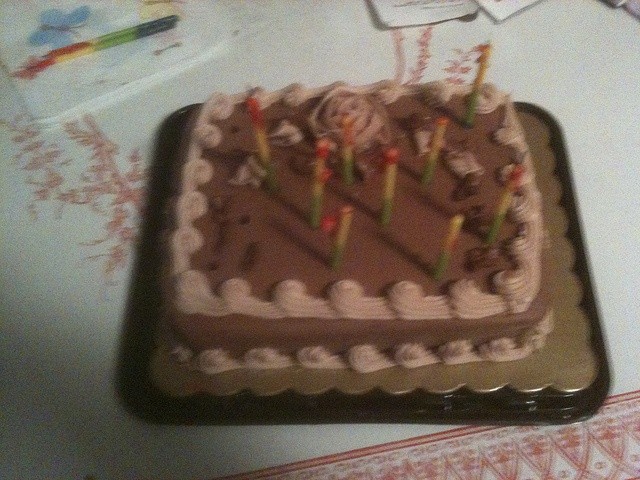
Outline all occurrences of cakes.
[156,26,555,375]
